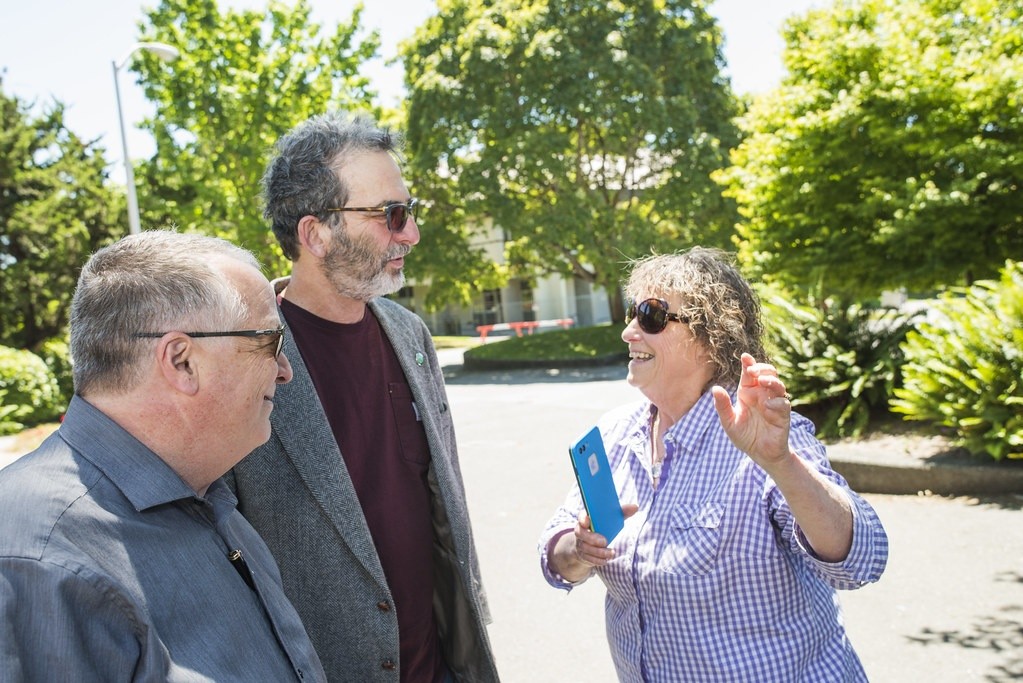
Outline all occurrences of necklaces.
[649,447,666,479]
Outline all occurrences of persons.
[1,228,329,683]
[222,110,504,683]
[538,246,888,683]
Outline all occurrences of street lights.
[112,42,178,233]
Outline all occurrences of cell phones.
[569,425,624,545]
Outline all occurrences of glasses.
[296,199,418,234]
[131,323,287,361]
[624,298,697,334]
